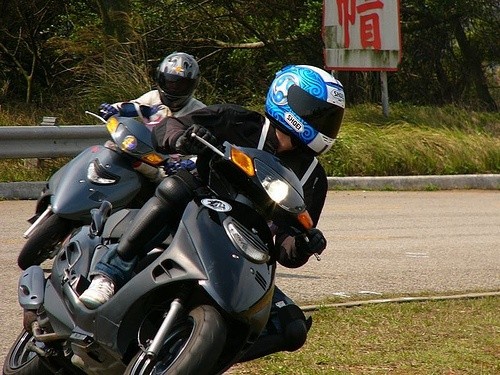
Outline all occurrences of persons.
[99,51,208,210]
[79,64,346,368]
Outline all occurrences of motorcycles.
[16,103,191,270]
[3,133,322,375]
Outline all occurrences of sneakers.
[79,276,115,309]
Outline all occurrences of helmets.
[263,63,346,155]
[156,52,199,111]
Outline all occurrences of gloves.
[169,124,217,156]
[292,228,327,261]
[163,158,196,177]
[98,101,119,120]
